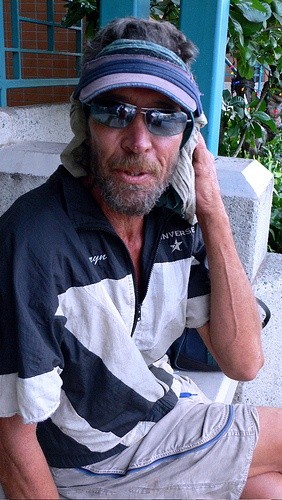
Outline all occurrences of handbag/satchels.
[167,327,221,373]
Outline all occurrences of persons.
[0,17,282,500]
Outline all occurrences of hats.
[74,54,203,115]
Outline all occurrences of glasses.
[82,99,192,137]
